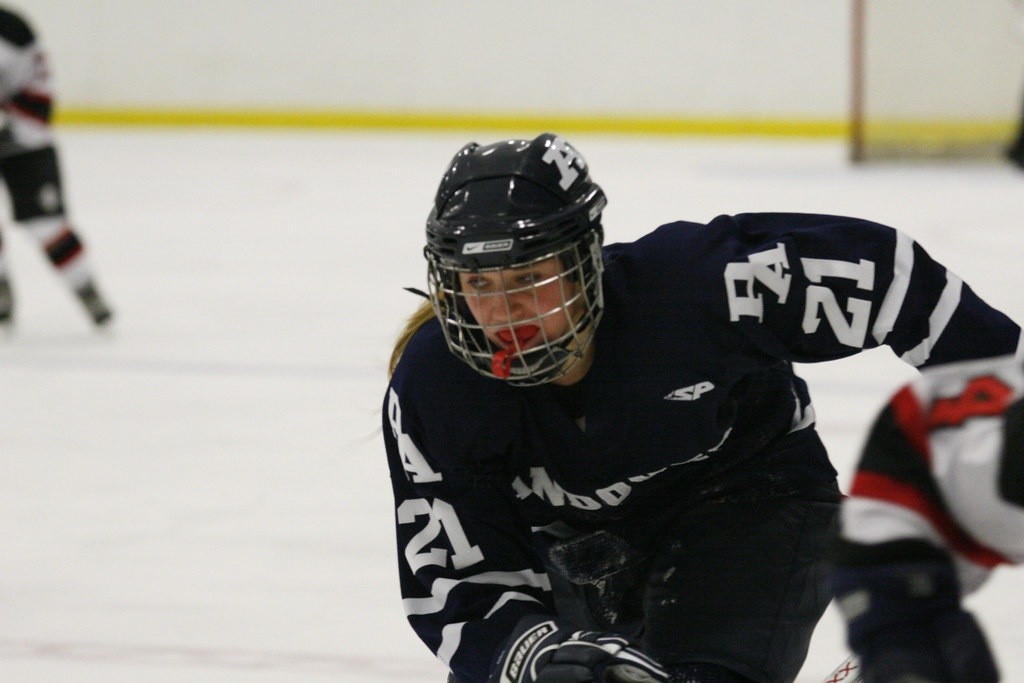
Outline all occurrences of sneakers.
[78,286,111,325]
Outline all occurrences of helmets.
[423,133,609,387]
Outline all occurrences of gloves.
[826,537,1001,683]
[488,615,672,683]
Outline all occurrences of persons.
[381,133,1024,683]
[0,6,113,324]
